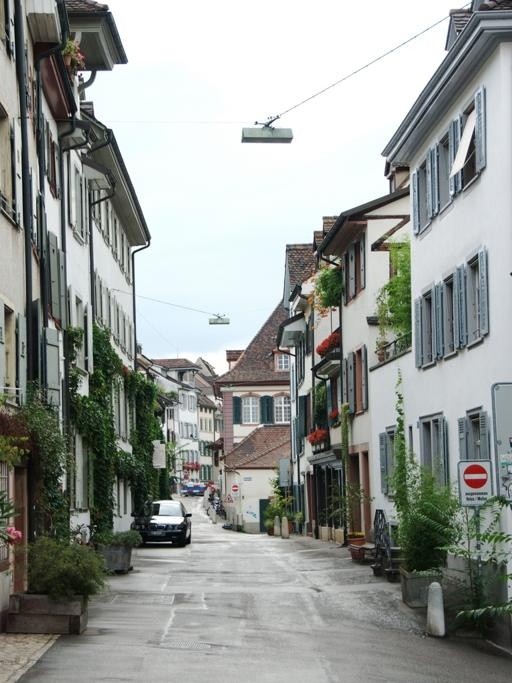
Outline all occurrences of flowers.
[63,32,88,83]
[302,262,345,323]
[0,490,22,556]
[315,329,345,359]
[307,426,331,447]
[345,530,366,540]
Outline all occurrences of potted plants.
[264,518,279,536]
[93,527,145,578]
[375,303,392,363]
[18,540,102,631]
[327,475,375,564]
[393,467,461,613]
[448,472,509,652]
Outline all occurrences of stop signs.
[232,485,238,491]
[463,465,489,489]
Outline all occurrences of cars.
[170,476,208,495]
[131,500,192,545]
